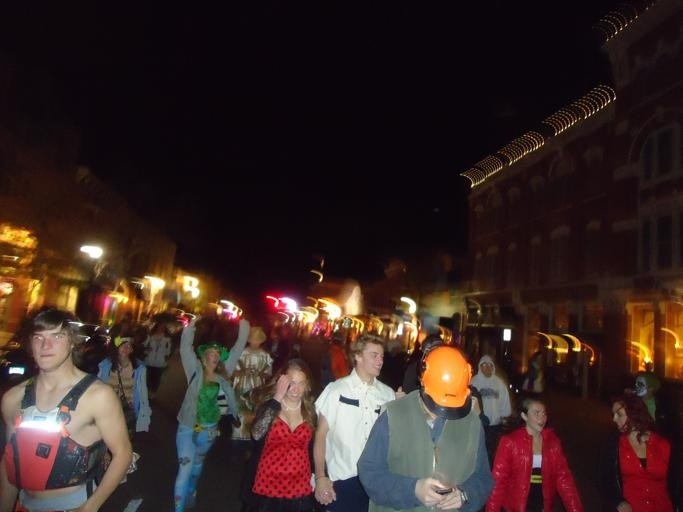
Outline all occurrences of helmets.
[417,346,473,421]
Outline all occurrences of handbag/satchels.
[117,390,136,429]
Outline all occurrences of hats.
[197,341,230,361]
[247,326,266,344]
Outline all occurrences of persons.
[1,302,682,512]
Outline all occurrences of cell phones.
[435,488,452,494]
